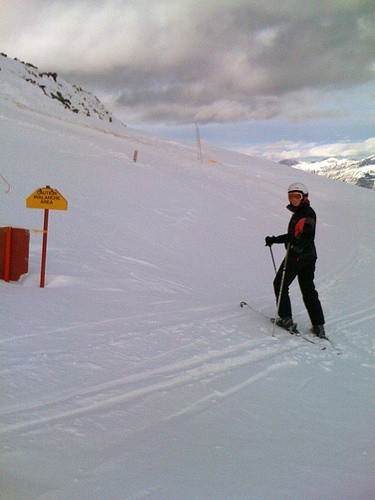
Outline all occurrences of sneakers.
[310,323,326,339]
[270,316,292,327]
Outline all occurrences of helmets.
[287,183,309,208]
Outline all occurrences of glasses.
[288,193,303,200]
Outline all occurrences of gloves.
[265,236,276,246]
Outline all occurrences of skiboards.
[240,301,341,357]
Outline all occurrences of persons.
[262,183,332,335]
